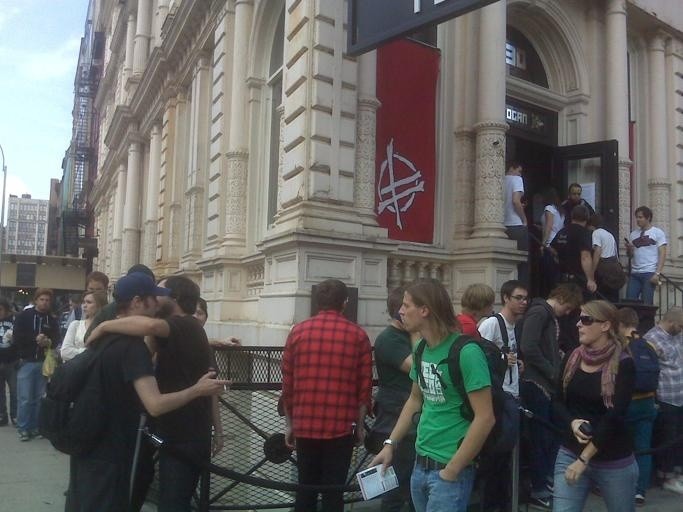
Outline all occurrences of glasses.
[576,316,605,325]
[509,295,528,303]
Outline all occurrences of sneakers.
[663,481,683,494]
[20,430,29,442]
[633,493,645,505]
[528,481,554,510]
[32,429,43,439]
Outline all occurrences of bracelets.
[383,439,400,450]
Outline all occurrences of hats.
[116,272,172,296]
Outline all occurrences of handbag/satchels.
[43,339,57,376]
[595,255,627,291]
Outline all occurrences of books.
[356,464,400,501]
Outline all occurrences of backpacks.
[416,337,507,460]
[42,333,121,456]
[627,332,659,392]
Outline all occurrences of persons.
[454,283,495,337]
[480,160,683,512]
[277,279,374,512]
[353,276,497,512]
[364,283,422,511]
[0,263,233,512]
